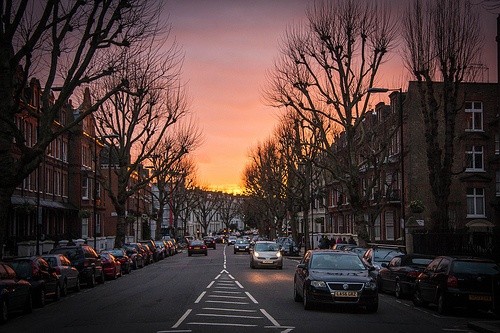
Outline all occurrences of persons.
[66,238,76,246]
[297,233,303,252]
[337,236,342,244]
[84,238,87,244]
[319,235,330,249]
[348,235,357,246]
[329,237,336,249]
[341,237,347,244]
[53,239,61,248]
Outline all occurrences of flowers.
[407,197,427,215]
[78,208,91,219]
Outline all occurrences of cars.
[248,240,285,270]
[39,253,81,297]
[0,254,62,309]
[379,254,438,300]
[121,236,179,270]
[412,253,500,320]
[227,235,237,246]
[203,235,217,250]
[294,248,379,312]
[92,247,122,281]
[186,239,208,256]
[182,227,408,272]
[106,246,134,274]
[232,237,252,254]
[0,259,33,326]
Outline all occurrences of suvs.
[47,244,105,288]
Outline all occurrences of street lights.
[93,134,120,253]
[366,87,405,245]
[296,162,313,250]
[36,86,63,256]
[136,164,155,243]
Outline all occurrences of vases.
[412,207,425,213]
[83,215,90,218]
